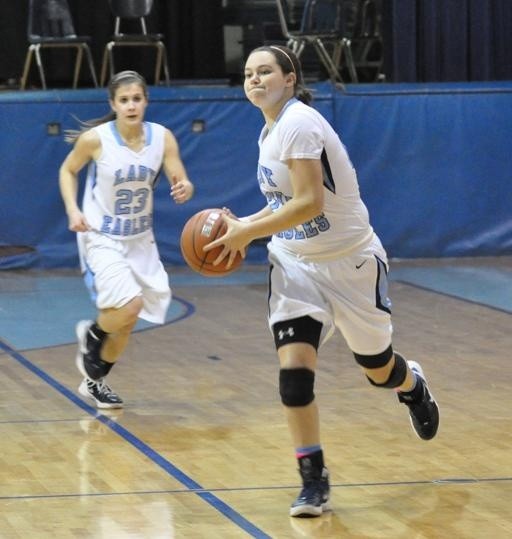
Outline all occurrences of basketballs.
[181,208,247,275]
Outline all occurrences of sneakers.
[397,360,440,441]
[290,465,332,518]
[75,320,106,385]
[77,378,124,410]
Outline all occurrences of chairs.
[276,0,387,81]
[19,0,99,90]
[100,1,171,90]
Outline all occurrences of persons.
[200,43,441,519]
[57,69,195,412]
